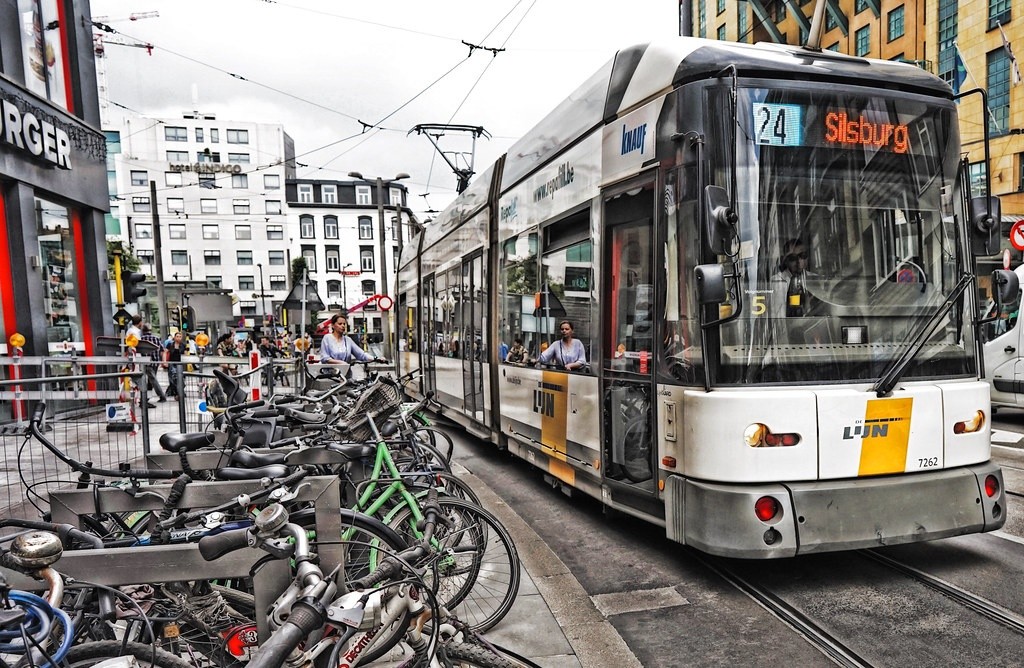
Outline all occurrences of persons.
[504,339,530,366]
[259,336,290,386]
[235,335,253,358]
[292,332,314,365]
[126,316,166,409]
[769,239,824,317]
[162,333,200,400]
[319,315,386,381]
[530,321,586,373]
[216,333,243,374]
[277,331,292,356]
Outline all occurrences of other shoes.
[139,401,156,408]
[158,396,166,402]
[174,395,179,401]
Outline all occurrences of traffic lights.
[120,271,148,303]
[171,306,183,333]
[180,307,197,333]
[361,326,367,333]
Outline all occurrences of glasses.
[785,250,810,263]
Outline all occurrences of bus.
[389,34,1006,559]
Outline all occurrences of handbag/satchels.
[161,342,172,369]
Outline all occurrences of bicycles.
[0,356,534,668]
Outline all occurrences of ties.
[798,274,805,306]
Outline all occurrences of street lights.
[342,263,352,327]
[348,168,412,363]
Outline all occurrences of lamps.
[32,255,40,268]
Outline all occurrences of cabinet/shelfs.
[45,264,72,328]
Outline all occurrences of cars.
[975,262,1024,411]
